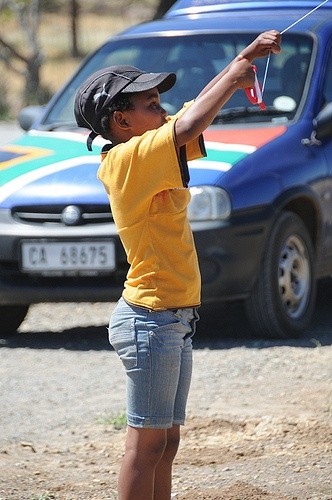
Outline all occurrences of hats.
[74,64,176,151]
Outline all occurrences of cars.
[1,0,332,338]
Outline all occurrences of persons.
[74,29,280,500]
